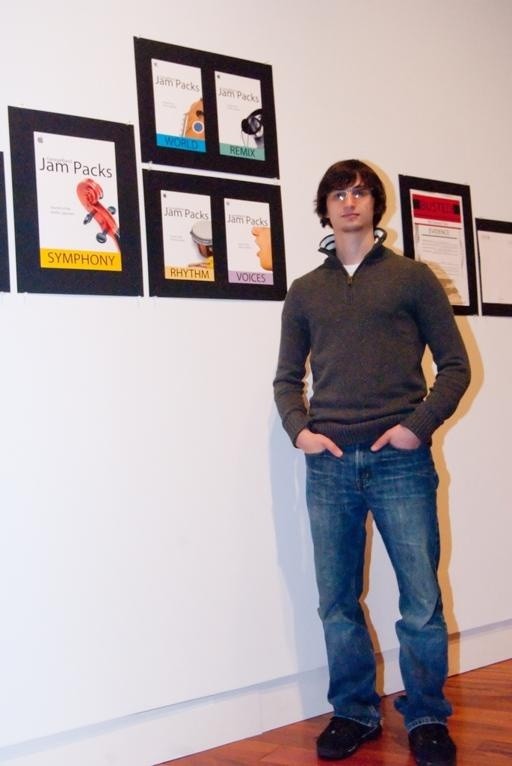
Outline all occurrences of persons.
[270,159,472,765]
[252,227,273,271]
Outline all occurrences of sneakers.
[409,723,457,766]
[317,717,382,760]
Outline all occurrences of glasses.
[325,189,371,202]
